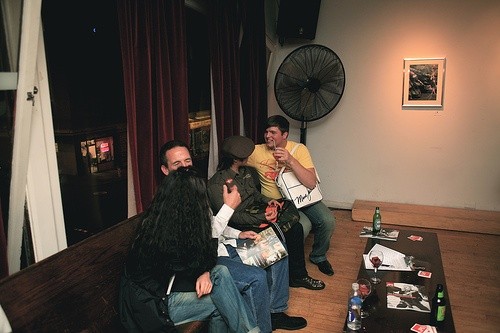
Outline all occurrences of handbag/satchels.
[262,197,300,232]
[275,143,323,208]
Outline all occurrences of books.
[386,281,431,312]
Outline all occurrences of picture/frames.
[401,58,445,107]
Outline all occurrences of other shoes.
[271,312,307,331]
[309,256,334,276]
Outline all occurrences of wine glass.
[356,279,372,318]
[369,250,384,284]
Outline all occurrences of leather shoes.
[289,276,325,290]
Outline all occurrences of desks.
[341,230,455,333]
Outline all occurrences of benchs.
[0,214,313,333]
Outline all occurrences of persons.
[206,137,326,291]
[120,166,265,333]
[220,115,334,276]
[158,139,308,333]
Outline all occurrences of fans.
[275,44,346,145]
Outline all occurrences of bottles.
[431,284,447,323]
[373,206,381,235]
[347,283,362,331]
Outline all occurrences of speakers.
[275,0,321,41]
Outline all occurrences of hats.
[222,135,255,159]
